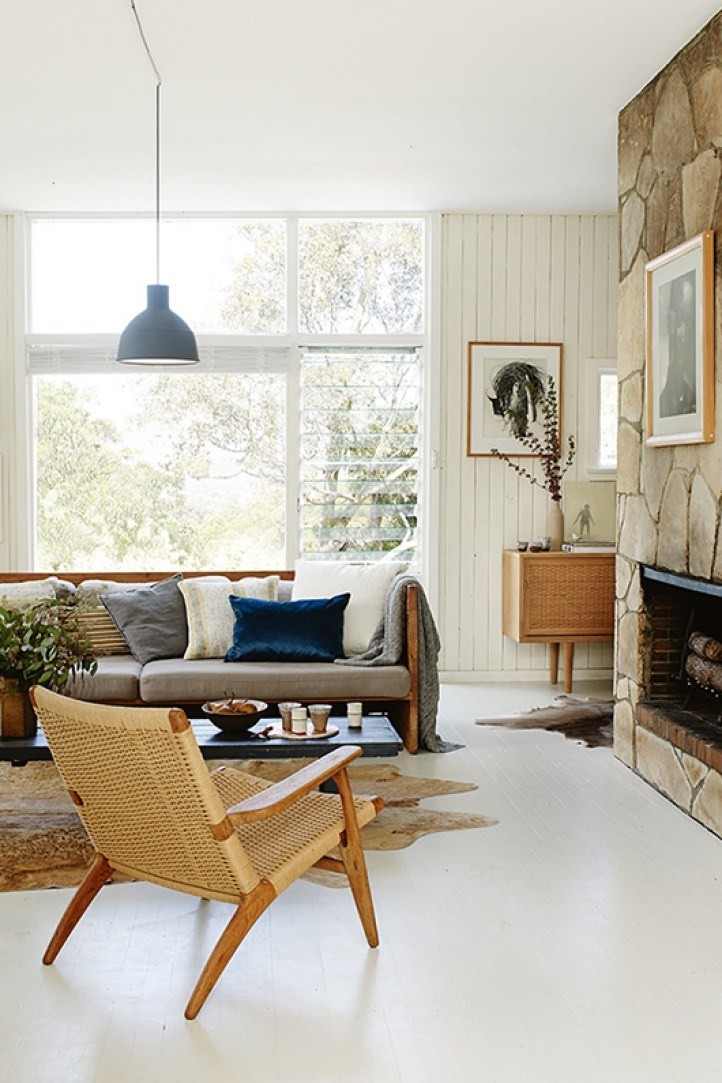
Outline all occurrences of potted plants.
[0,584,110,740]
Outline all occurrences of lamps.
[118,79,202,371]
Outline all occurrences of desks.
[499,545,615,694]
[0,712,407,795]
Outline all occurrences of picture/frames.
[466,340,564,460]
[582,355,619,485]
[641,227,718,450]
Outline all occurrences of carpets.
[0,762,502,895]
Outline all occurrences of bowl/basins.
[201,699,269,733]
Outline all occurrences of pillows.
[95,571,184,664]
[72,574,158,604]
[175,572,281,661]
[225,591,351,664]
[0,575,61,637]
[241,575,296,604]
[290,556,413,654]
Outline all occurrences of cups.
[346,702,362,727]
[308,704,332,732]
[539,537,551,551]
[530,541,541,553]
[291,707,307,733]
[278,702,301,731]
[518,542,528,552]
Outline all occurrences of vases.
[546,495,565,551]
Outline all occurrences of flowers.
[489,373,579,503]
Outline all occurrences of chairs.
[27,677,389,1021]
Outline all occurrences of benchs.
[0,567,422,755]
